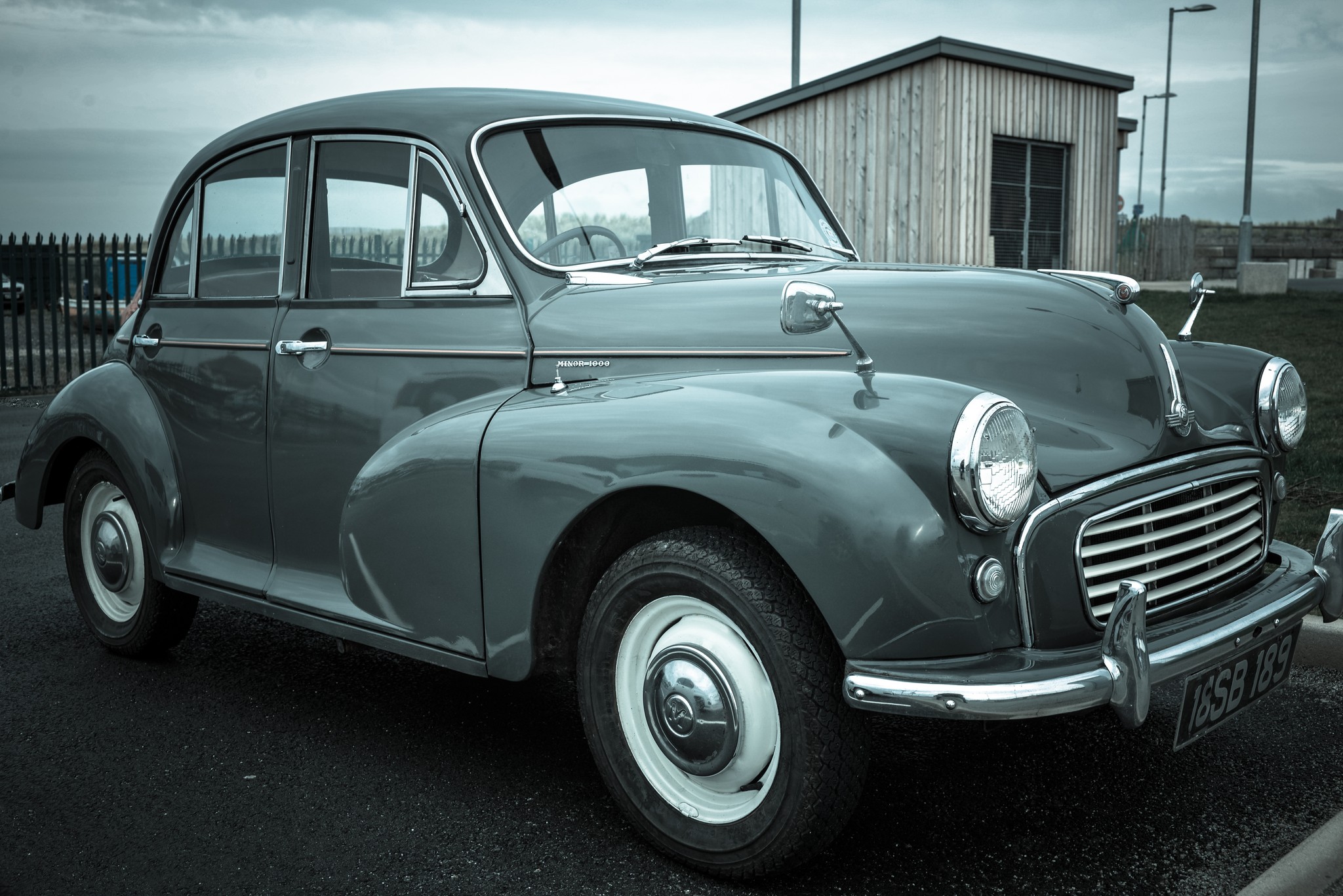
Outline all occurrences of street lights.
[1157,3,1216,275]
[1134,92,1178,283]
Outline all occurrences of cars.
[0,79,1343,886]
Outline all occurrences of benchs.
[158,269,463,296]
